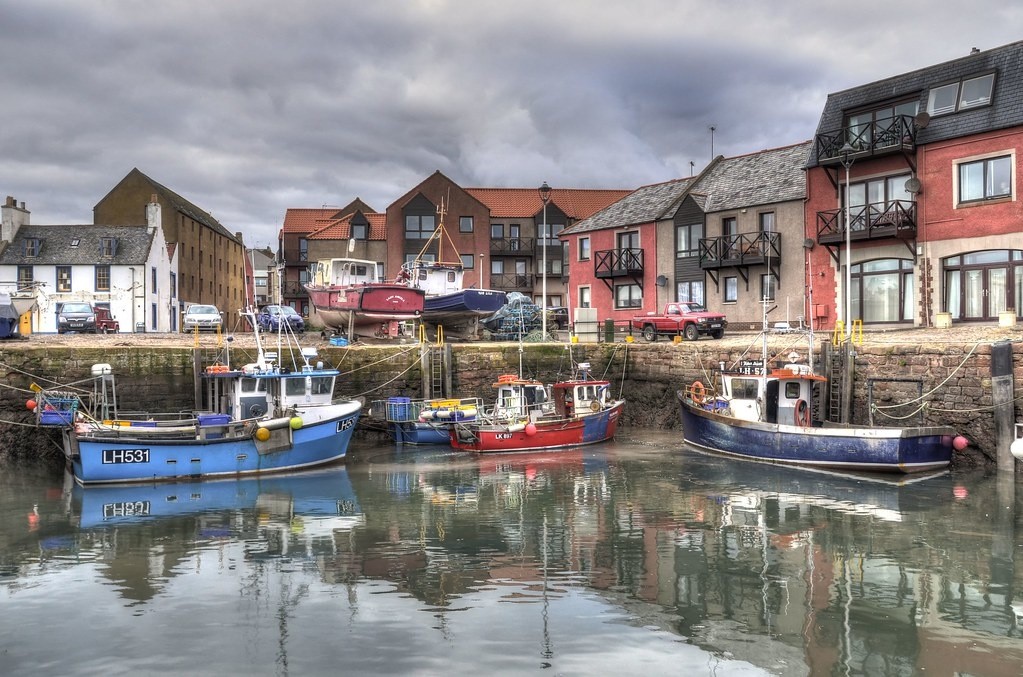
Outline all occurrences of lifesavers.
[207,365,228,374]
[690,381,705,403]
[497,374,518,382]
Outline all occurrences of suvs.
[93,306,120,334]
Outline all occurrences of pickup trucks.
[631,302,727,341]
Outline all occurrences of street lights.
[479,255,484,291]
[537,180,553,342]
[838,142,860,342]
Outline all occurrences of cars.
[55,303,100,334]
[258,305,306,335]
[182,302,224,334]
[541,306,568,329]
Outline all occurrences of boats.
[392,185,506,341]
[303,255,426,341]
[673,440,951,676]
[449,295,625,453]
[32,213,366,485]
[675,210,958,477]
[0,278,42,340]
[36,462,368,566]
[384,398,490,447]
